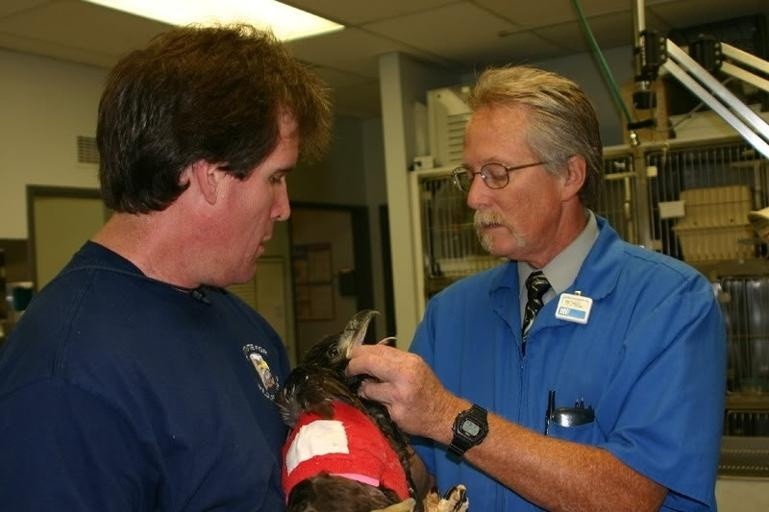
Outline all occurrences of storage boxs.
[673,185,761,266]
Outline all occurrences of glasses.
[453,158,552,194]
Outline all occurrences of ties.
[520,270,551,358]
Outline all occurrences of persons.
[0,22,332,512]
[344,63,728,512]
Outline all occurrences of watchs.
[446,404,488,464]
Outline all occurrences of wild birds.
[274,309,471,512]
